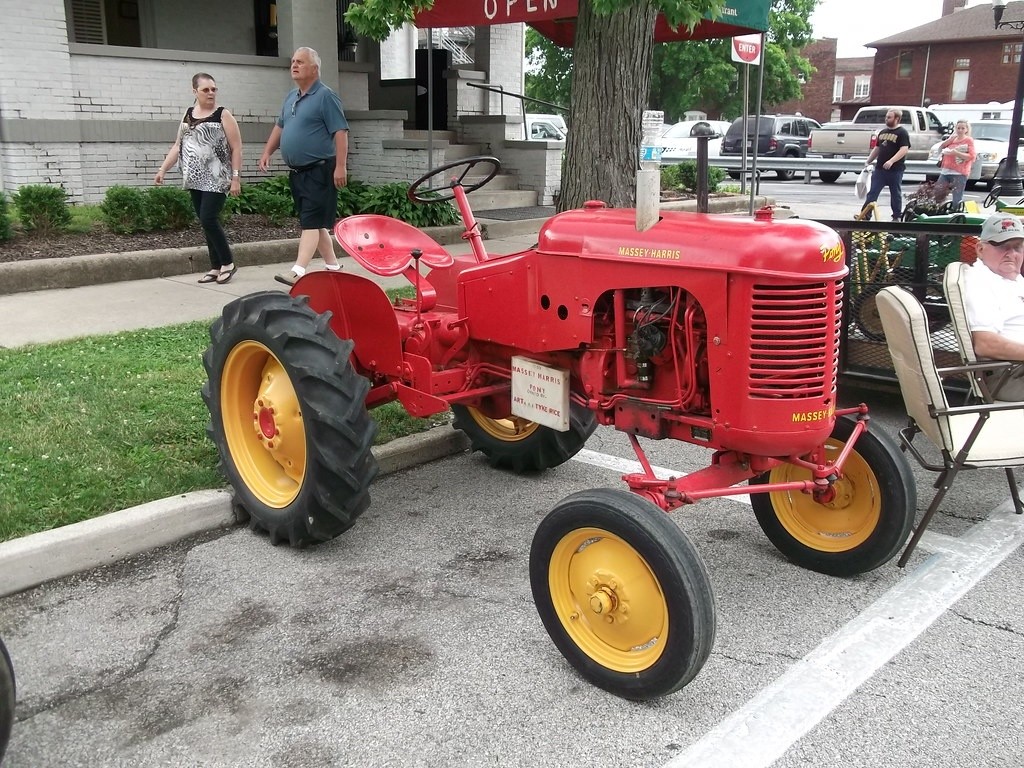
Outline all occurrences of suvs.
[718,113,823,180]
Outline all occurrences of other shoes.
[274,271,300,286]
[324,265,344,273]
[854,214,866,220]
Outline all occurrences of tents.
[415,0,771,216]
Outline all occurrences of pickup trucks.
[804,106,954,183]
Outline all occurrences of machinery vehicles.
[198,119,917,702]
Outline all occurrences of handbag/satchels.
[854,166,871,200]
[937,154,944,167]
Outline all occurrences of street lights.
[989,0,1024,197]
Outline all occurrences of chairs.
[942,259,1024,403]
[875,287,1024,568]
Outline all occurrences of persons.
[963,210,1024,401]
[259,47,350,287]
[154,72,242,283]
[853,107,911,221]
[926,119,976,211]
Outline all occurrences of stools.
[336,213,453,314]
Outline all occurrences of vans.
[526,113,569,142]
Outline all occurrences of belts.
[295,159,326,172]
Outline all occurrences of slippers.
[216,264,237,283]
[197,273,217,283]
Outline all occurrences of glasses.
[198,87,218,93]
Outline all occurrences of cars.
[658,119,733,155]
[926,118,1024,190]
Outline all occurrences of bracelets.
[232,169,241,177]
[158,168,167,173]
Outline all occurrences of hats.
[981,212,1024,243]
